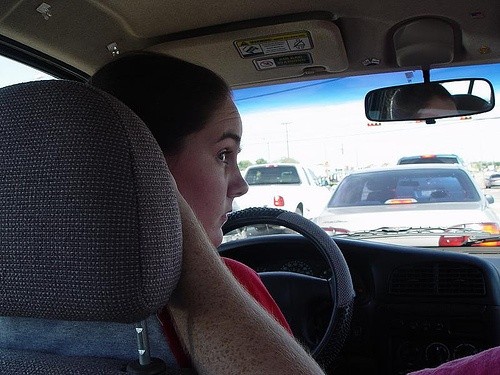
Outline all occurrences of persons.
[165,167,500,375]
[85,50,294,375]
[389,83,458,122]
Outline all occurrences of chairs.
[0,80,184,375]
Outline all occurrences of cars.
[316,154,500,260]
[228,162,332,222]
